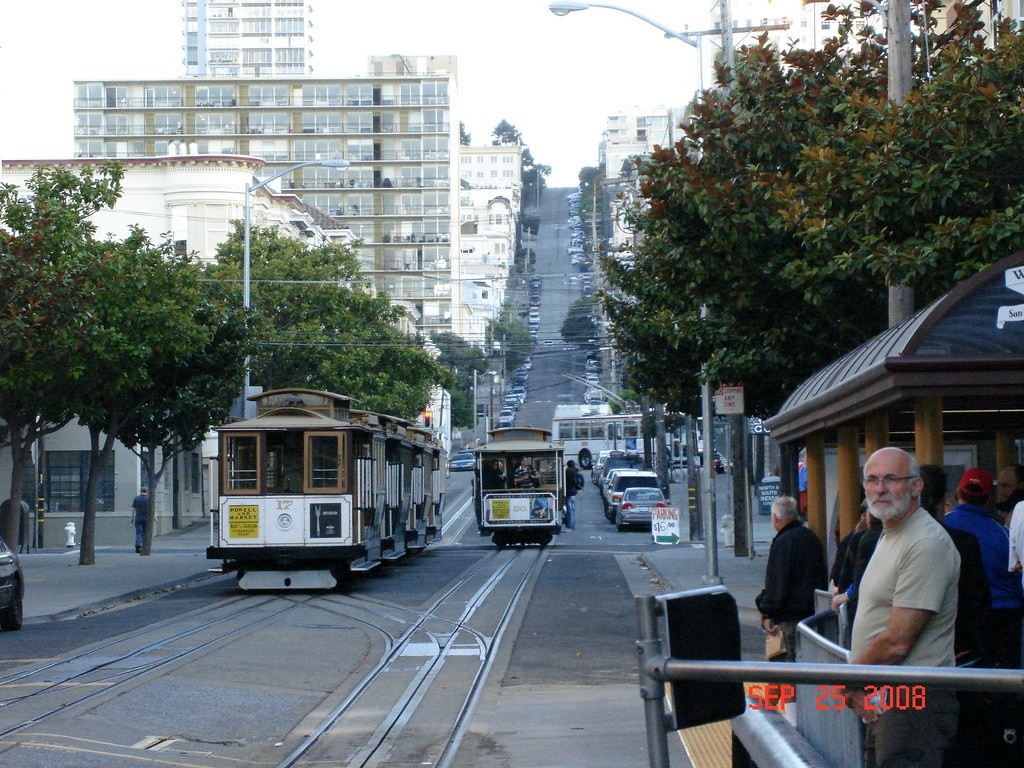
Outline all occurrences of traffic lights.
[424,410,431,428]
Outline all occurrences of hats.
[141,487,147,492]
[960,468,992,495]
[567,460,574,466]
[860,499,869,509]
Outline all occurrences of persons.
[514,456,539,493]
[482,459,506,493]
[920,465,1024,768]
[130,487,157,553]
[284,458,304,491]
[832,497,883,631]
[565,460,578,530]
[840,447,961,768]
[754,496,828,712]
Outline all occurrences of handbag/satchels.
[765,625,787,659]
[532,467,540,488]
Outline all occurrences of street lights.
[547,0,723,587]
[472,369,497,449]
[237,157,351,426]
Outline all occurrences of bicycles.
[668,460,686,484]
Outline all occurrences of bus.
[551,403,705,470]
[204,386,449,593]
[470,425,568,550]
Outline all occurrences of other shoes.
[137,546,141,553]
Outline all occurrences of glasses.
[494,464,499,466]
[997,482,1019,489]
[865,475,917,487]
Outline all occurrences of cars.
[449,452,475,472]
[495,186,606,430]
[590,449,669,534]
[0,536,26,632]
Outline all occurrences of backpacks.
[576,470,584,490]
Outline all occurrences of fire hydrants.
[63,521,77,548]
